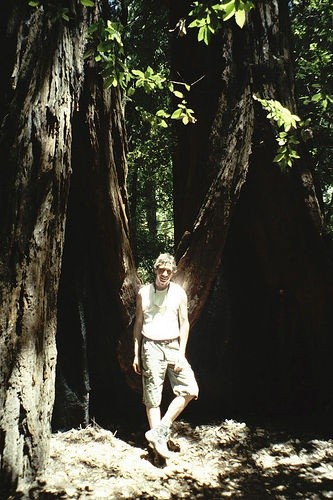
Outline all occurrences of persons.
[131,253,201,464]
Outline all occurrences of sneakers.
[145,422,180,458]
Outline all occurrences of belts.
[143,336,178,345]
